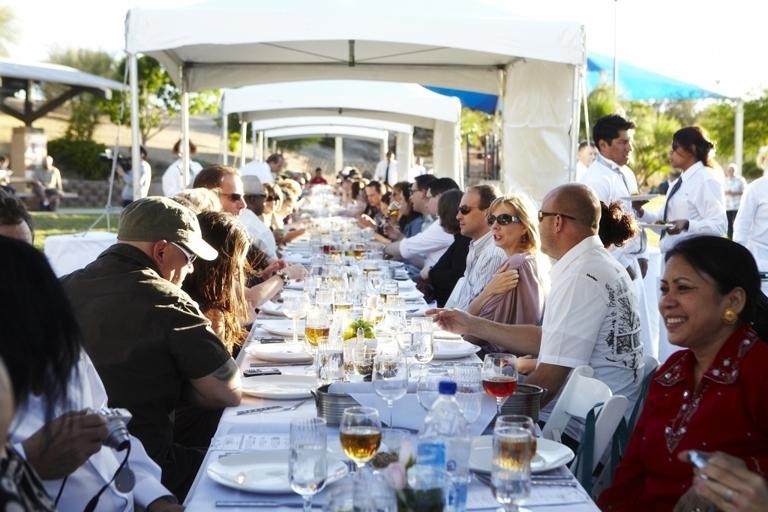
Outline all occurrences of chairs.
[541,364,628,504]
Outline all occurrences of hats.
[115,195,219,262]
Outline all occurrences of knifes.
[249,361,313,367]
[215,499,324,508]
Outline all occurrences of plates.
[260,320,306,339]
[261,232,308,316]
[619,194,659,202]
[467,435,574,474]
[640,220,676,230]
[208,447,346,493]
[244,343,313,362]
[406,340,480,359]
[240,375,328,399]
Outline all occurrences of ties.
[660,176,683,239]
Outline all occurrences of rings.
[724,489,734,499]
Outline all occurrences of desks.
[44,233,117,278]
[181,179,603,512]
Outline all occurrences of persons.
[335,169,459,282]
[635,125,728,368]
[1,235,82,512]
[176,187,308,360]
[235,153,313,264]
[597,236,768,511]
[56,195,245,502]
[115,144,151,209]
[0,188,184,511]
[182,212,251,356]
[726,166,745,239]
[425,182,644,478]
[0,155,16,196]
[464,191,547,359]
[193,165,287,315]
[418,189,473,308]
[376,152,397,187]
[732,146,767,298]
[162,138,204,197]
[579,114,650,279]
[444,184,509,313]
[32,156,64,211]
[678,450,768,512]
[579,141,597,178]
[310,167,326,184]
[599,202,661,367]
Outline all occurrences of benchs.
[10,177,78,210]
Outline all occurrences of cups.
[415,364,482,425]
[308,215,436,383]
[354,466,450,511]
[105,150,112,157]
[492,427,531,512]
[340,407,382,465]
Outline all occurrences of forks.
[236,400,305,416]
[477,475,577,490]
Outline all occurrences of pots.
[309,384,549,423]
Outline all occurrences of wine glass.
[379,201,400,227]
[483,352,517,416]
[288,417,328,512]
[372,353,411,451]
[282,292,311,344]
[496,414,539,511]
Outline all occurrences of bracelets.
[272,270,290,286]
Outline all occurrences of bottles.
[675,448,731,511]
[423,381,474,511]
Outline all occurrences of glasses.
[266,196,280,202]
[171,241,198,265]
[537,210,577,222]
[456,205,479,214]
[487,214,520,226]
[222,192,242,203]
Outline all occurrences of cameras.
[88,406,137,453]
